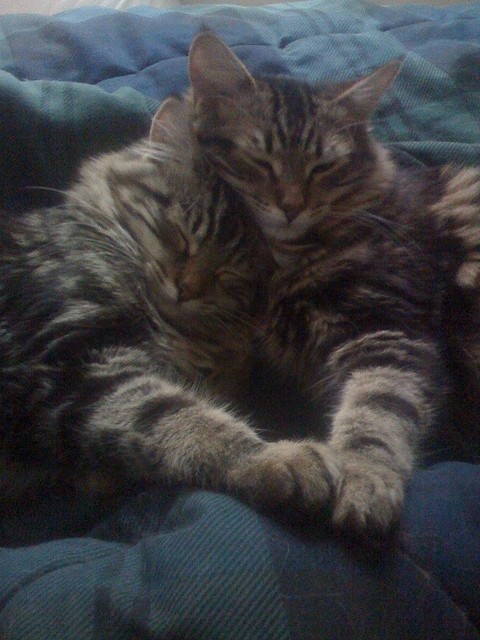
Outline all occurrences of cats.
[187,21,479,533]
[1,92,345,508]
